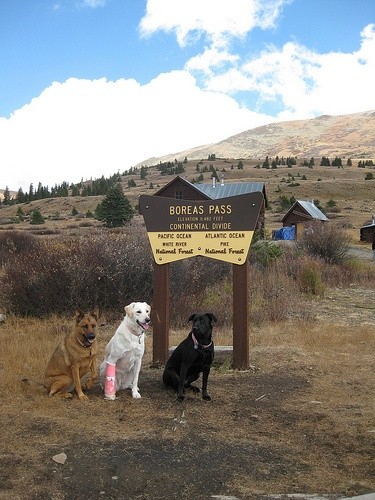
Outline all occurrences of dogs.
[22,305,99,402]
[99,301,152,401]
[162,311,218,404]
